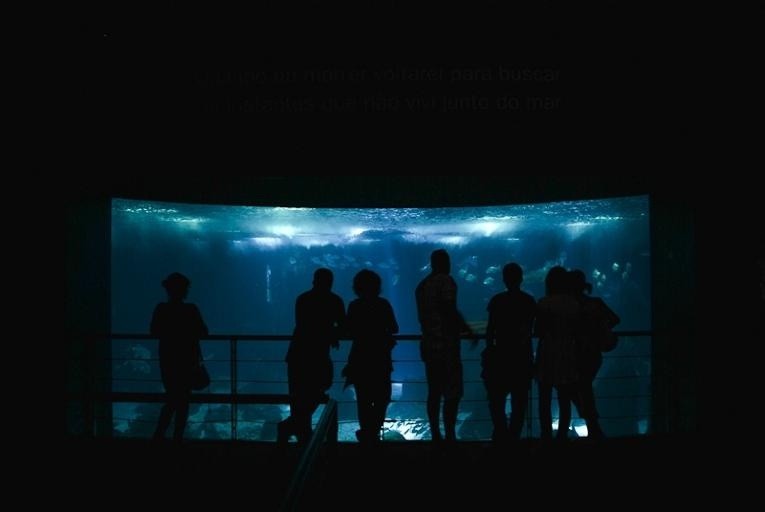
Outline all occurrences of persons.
[554,269,621,441]
[532,266,580,443]
[344,269,399,452]
[414,245,482,442]
[481,260,536,441]
[147,271,211,443]
[274,266,346,449]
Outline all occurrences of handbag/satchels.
[481,344,505,372]
[189,363,210,389]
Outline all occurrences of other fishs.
[421,263,433,273]
[455,256,634,291]
[287,252,401,286]
